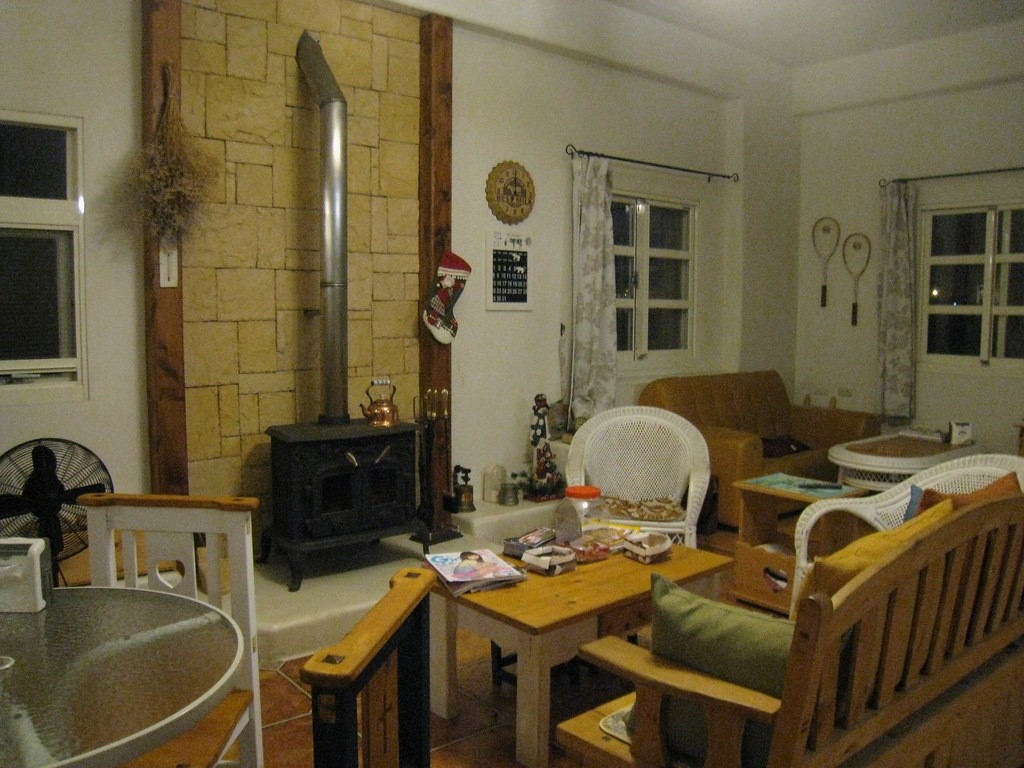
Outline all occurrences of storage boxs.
[360,378,399,429]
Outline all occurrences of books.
[424,548,526,599]
[578,517,641,556]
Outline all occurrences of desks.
[421,527,739,768]
[825,425,987,496]
[1,586,245,768]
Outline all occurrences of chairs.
[790,454,1023,620]
[78,492,265,768]
[566,404,713,551]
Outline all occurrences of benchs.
[556,488,1022,768]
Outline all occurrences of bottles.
[555,486,613,565]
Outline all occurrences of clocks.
[486,160,537,227]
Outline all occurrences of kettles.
[359,379,400,429]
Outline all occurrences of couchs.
[642,371,880,529]
